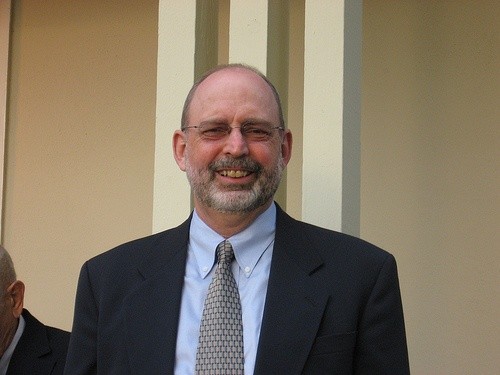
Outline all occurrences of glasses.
[181,123,283,142]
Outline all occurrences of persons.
[0,244,71,375]
[63,64,411,375]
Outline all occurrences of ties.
[194,240,244,375]
